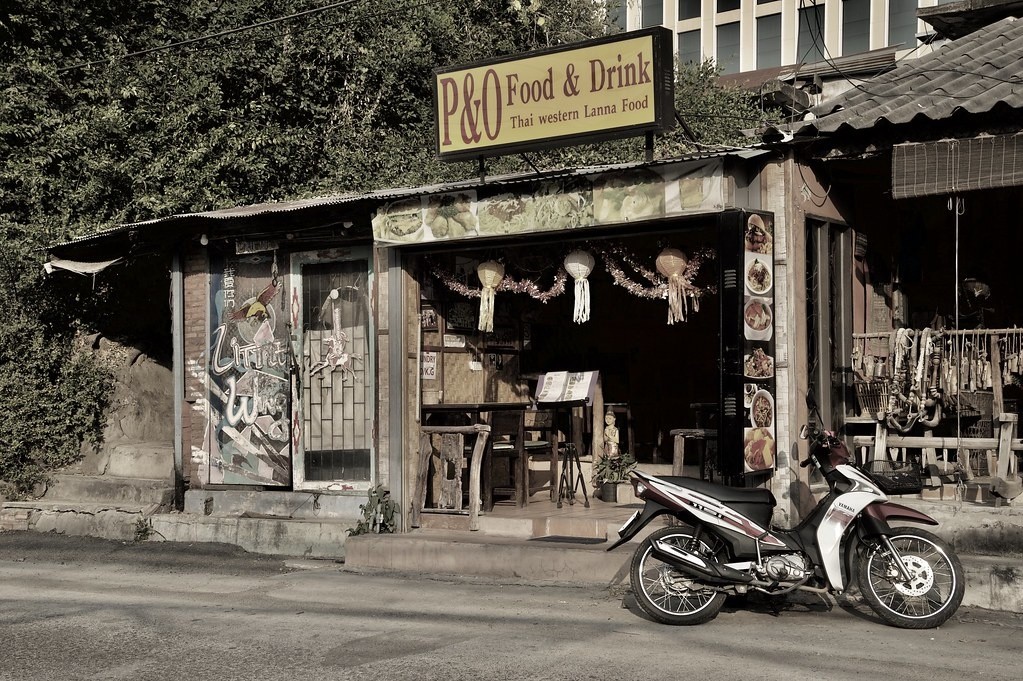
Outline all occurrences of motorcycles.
[606,383,965,631]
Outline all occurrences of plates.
[750,389,774,427]
[744,427,776,474]
[744,320,773,341]
[746,259,772,295]
[744,384,758,409]
[744,355,774,379]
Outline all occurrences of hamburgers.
[385,199,422,236]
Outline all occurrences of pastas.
[478,192,531,234]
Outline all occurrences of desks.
[571,403,644,460]
[420,402,527,511]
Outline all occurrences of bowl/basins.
[745,384,753,394]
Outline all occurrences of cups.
[744,298,772,334]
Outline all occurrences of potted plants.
[588,453,638,501]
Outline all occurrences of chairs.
[463,407,559,503]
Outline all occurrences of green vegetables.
[532,175,593,228]
